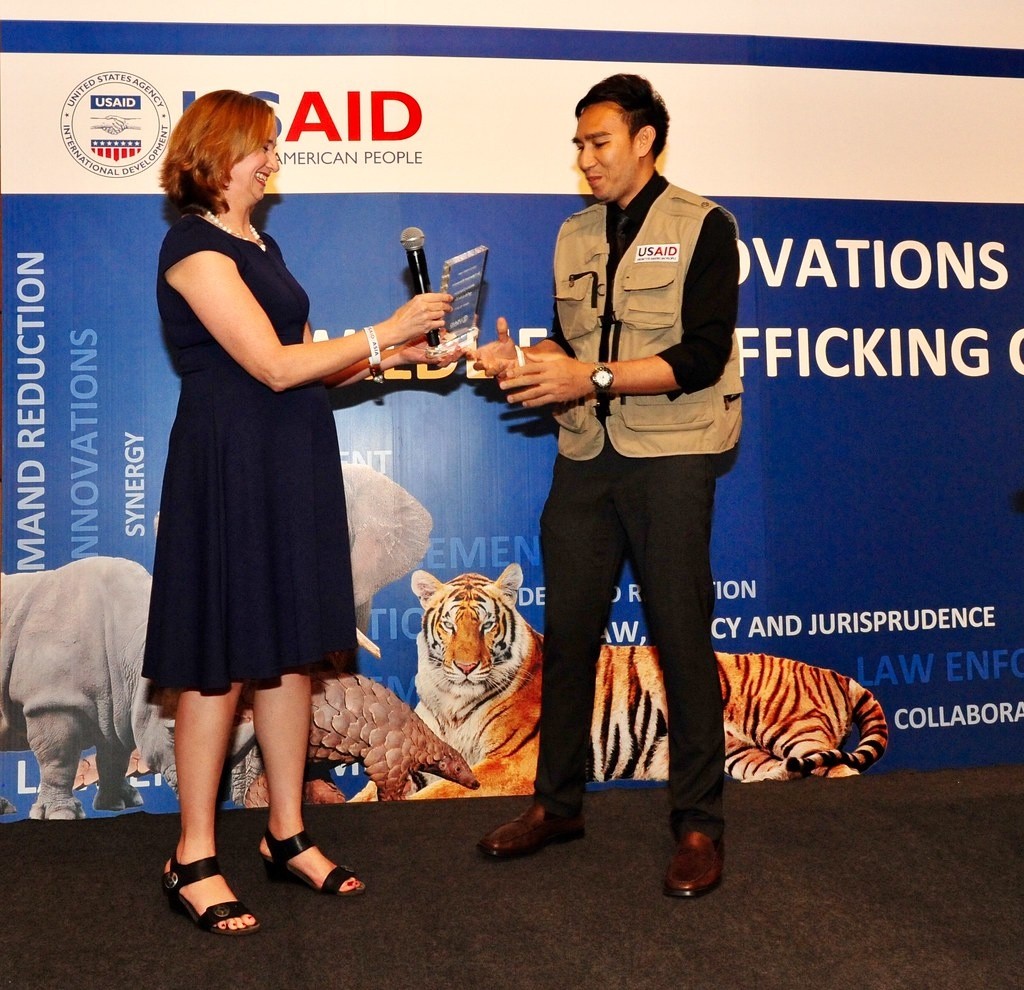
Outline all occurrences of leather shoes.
[477,802,582,860]
[664,824,725,896]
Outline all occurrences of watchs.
[590,362,614,392]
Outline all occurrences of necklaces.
[206,209,265,254]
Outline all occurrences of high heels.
[160,853,262,936]
[259,827,366,897]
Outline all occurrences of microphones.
[399,226,441,347]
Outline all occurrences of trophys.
[425,244,489,360]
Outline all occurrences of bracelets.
[369,357,384,383]
[515,345,525,366]
[364,326,382,365]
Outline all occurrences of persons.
[141,88,469,936]
[469,74,743,895]
